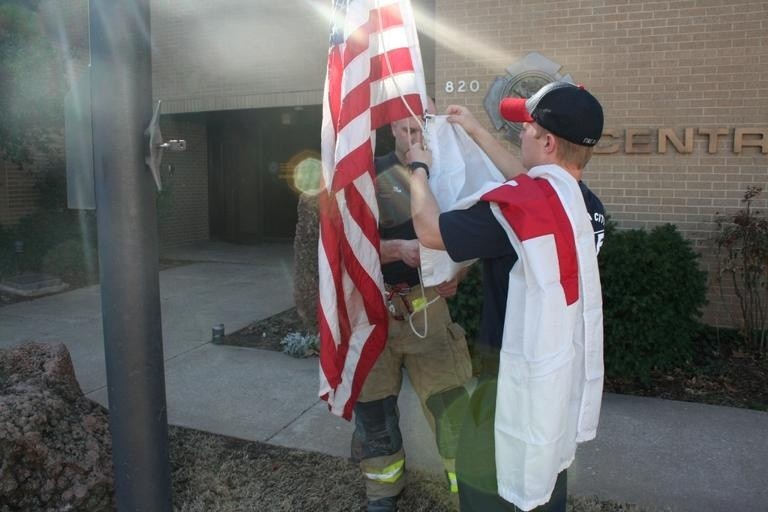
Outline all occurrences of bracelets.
[403,161,430,180]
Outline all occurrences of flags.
[316,0,427,423]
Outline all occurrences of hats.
[498,80,605,148]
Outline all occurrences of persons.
[350,91,475,512]
[404,80,608,512]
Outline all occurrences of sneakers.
[367,483,407,512]
